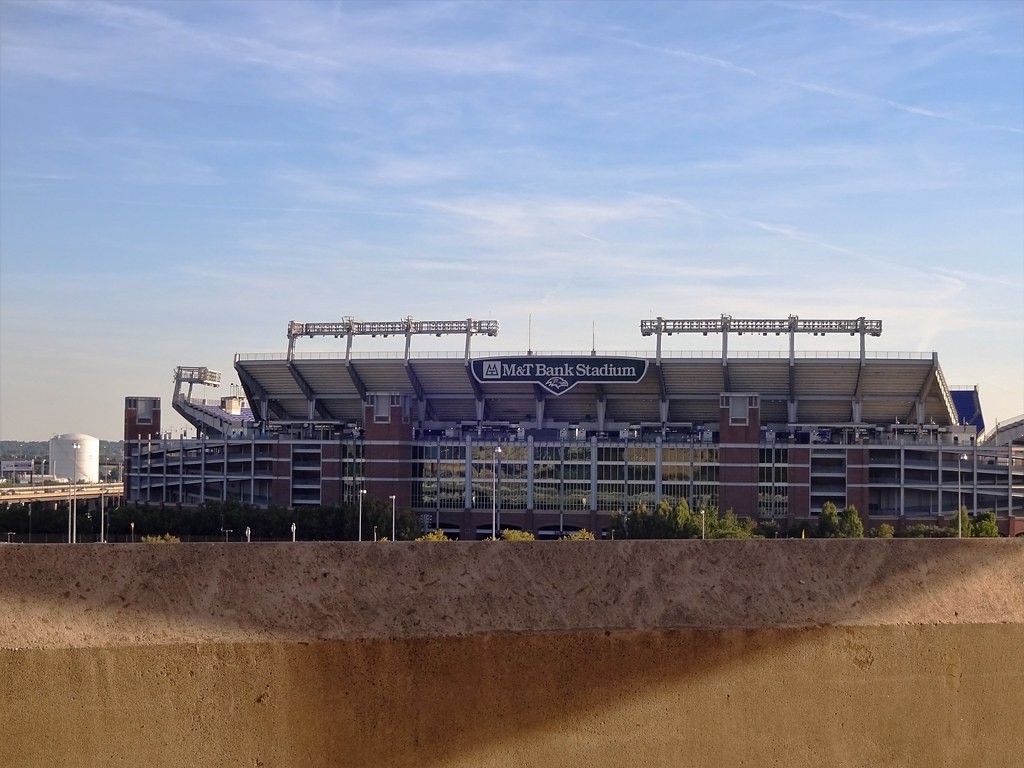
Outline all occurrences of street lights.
[359,489,367,541]
[492,446,502,542]
[373,526,377,541]
[12,454,17,483]
[103,446,106,465]
[957,452,968,538]
[42,459,47,486]
[130,522,135,542]
[611,529,616,540]
[699,508,706,540]
[105,458,110,483]
[388,495,396,541]
[72,441,81,544]
[67,477,73,543]
[98,479,104,543]
